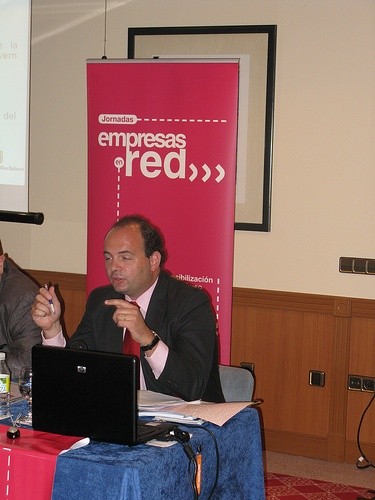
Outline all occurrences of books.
[137,400,211,425]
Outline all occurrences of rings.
[123,314,126,321]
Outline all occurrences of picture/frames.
[127,25,278,234]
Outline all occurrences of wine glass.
[18,366,33,423]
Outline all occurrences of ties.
[123,302,141,358]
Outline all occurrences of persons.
[0,240,44,381]
[30,214,226,404]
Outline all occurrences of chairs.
[218,364,256,402]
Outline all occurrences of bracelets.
[140,330,162,351]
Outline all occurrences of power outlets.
[362,376,375,393]
[339,256,375,275]
[348,374,362,391]
[239,361,255,373]
[309,370,325,388]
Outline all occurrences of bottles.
[0,352,11,420]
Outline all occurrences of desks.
[0,381,267,500]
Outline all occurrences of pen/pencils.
[44,284,55,315]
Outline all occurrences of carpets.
[264,471,375,500]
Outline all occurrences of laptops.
[31,345,179,447]
